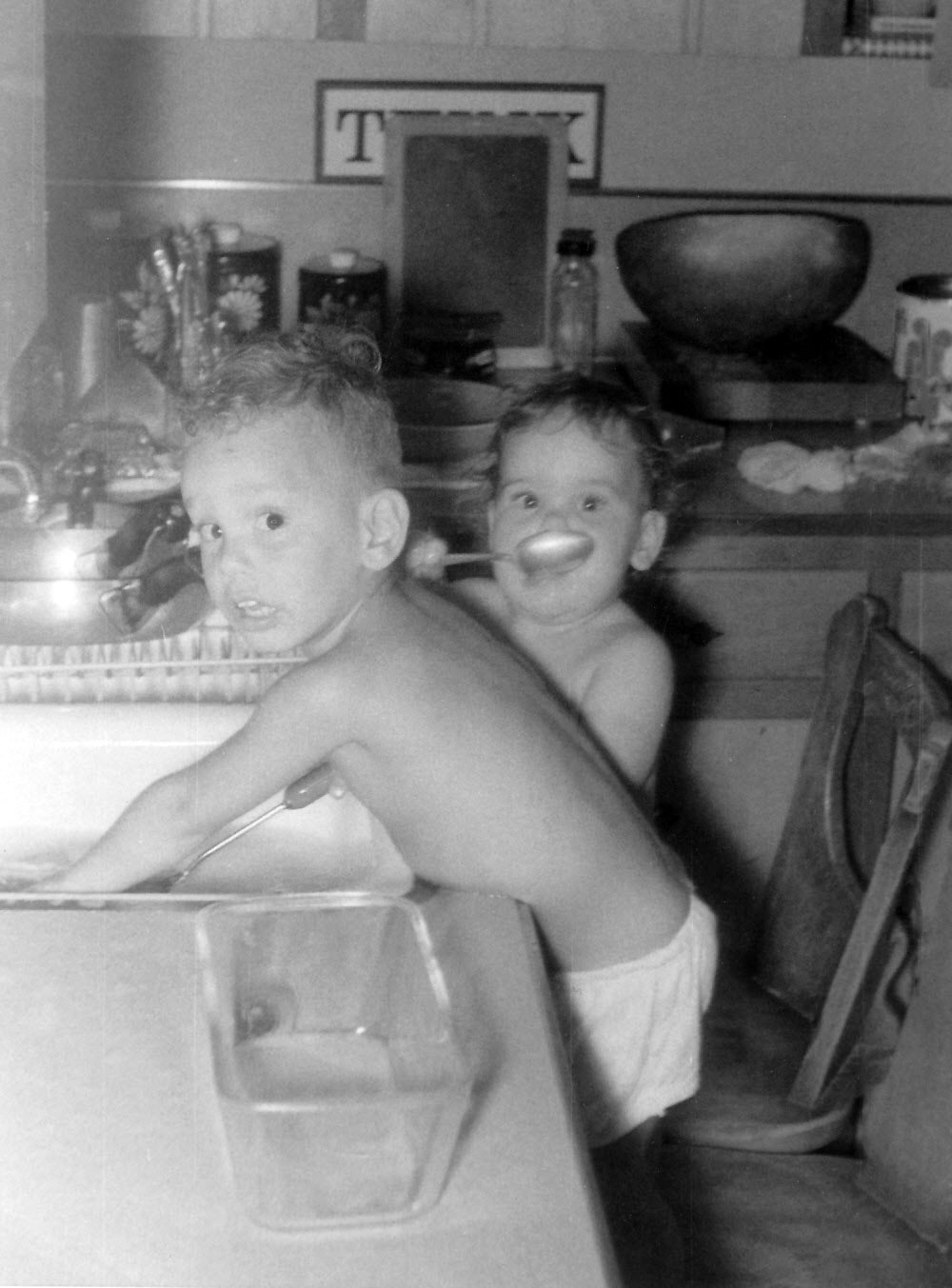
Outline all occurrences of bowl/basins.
[386,379,517,464]
[613,209,870,354]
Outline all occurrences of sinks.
[0,500,213,650]
[1,699,415,901]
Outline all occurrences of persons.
[27,327,723,1288]
[404,377,675,823]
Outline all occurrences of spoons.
[439,528,595,577]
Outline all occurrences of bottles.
[299,247,387,340]
[206,222,282,335]
[549,227,598,369]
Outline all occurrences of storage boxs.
[195,890,473,1230]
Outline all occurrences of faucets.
[1,451,43,505]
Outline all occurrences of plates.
[99,465,181,506]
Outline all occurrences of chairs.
[613,871,952,1288]
[657,591,952,1152]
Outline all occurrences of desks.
[0,889,620,1288]
